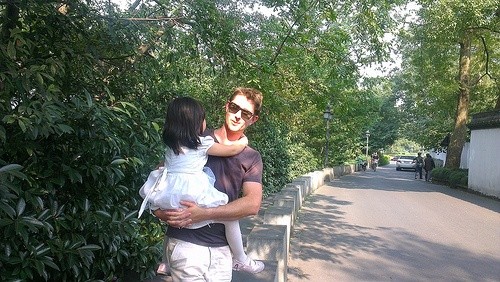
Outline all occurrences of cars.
[395,155,417,171]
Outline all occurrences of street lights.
[323,102,334,168]
[365,129,371,160]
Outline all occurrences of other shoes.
[155,262,171,276]
[231,256,265,274]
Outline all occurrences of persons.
[423,153,436,182]
[371,151,379,171]
[138,96,265,274]
[413,152,424,179]
[150,87,263,282]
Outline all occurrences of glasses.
[228,102,254,121]
